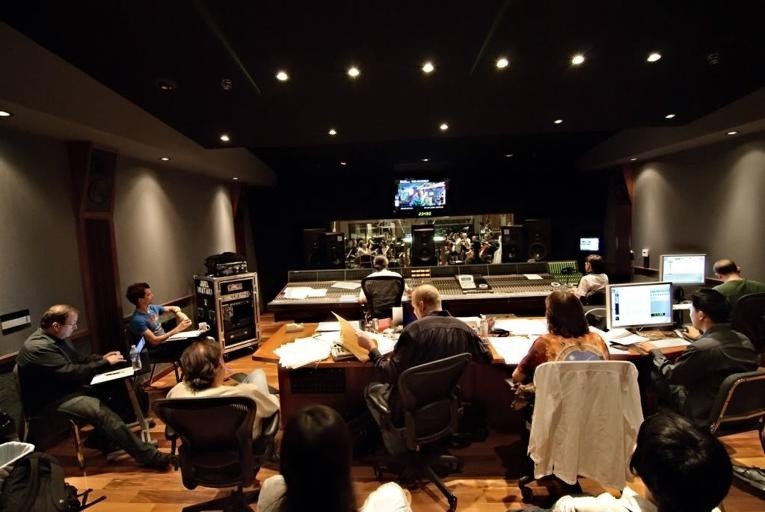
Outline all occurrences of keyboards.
[636,338,692,349]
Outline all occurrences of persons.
[353,283,494,479]
[571,254,609,300]
[711,258,765,304]
[126,283,205,361]
[511,291,611,381]
[257,404,412,512]
[165,340,281,442]
[635,290,759,429]
[345,215,500,304]
[552,409,733,512]
[16,303,173,472]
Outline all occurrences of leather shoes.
[144,450,171,470]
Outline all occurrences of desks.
[251,315,714,431]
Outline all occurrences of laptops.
[98,334,147,374]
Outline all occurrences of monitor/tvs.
[605,281,673,329]
[658,252,707,287]
[392,176,452,217]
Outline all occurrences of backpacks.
[0,452,80,512]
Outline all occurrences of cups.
[199,322,210,330]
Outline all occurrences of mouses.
[680,328,688,334]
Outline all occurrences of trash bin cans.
[0,442,35,482]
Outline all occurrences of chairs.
[150,395,279,511]
[504,359,645,500]
[645,355,765,434]
[366,352,484,506]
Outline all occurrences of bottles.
[480,315,488,339]
[129,345,143,372]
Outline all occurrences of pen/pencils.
[105,371,120,375]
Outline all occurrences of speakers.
[524,219,547,261]
[303,228,325,270]
[321,231,346,269]
[500,224,524,263]
[410,224,438,266]
